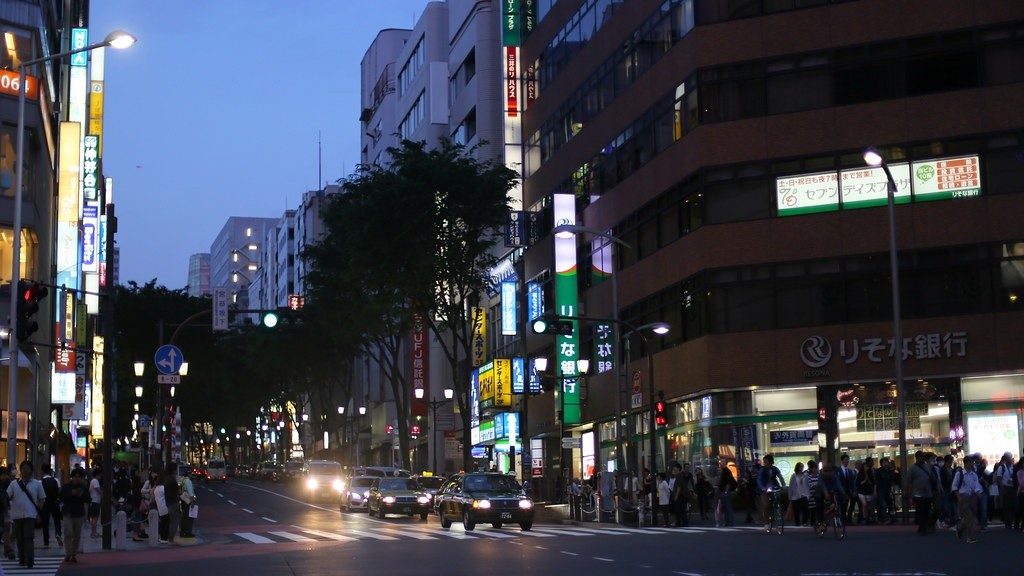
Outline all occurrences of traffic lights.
[531,318,573,336]
[655,401,668,426]
[18,280,48,342]
[261,310,311,333]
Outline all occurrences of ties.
[845,469,848,480]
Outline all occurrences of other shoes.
[967,538,978,543]
[180,533,195,537]
[56,534,63,545]
[40,544,48,548]
[132,537,144,541]
[956,531,963,543]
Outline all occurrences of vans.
[299,458,343,500]
[207,459,227,482]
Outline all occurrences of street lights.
[338,406,367,465]
[622,322,672,524]
[863,146,909,523]
[554,223,632,523]
[415,386,454,476]
[7,30,140,468]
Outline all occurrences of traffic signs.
[561,437,580,443]
[562,443,581,449]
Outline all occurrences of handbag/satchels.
[180,491,195,504]
[188,505,199,518]
[784,501,794,521]
[948,493,958,504]
[929,478,940,494]
[953,502,972,530]
[989,482,999,495]
[669,488,674,505]
[139,497,148,512]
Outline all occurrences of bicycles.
[764,487,785,536]
[814,490,847,538]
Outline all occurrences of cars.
[433,473,535,532]
[234,459,303,482]
[342,467,448,520]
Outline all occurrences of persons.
[571,450,1024,544]
[0,459,198,568]
